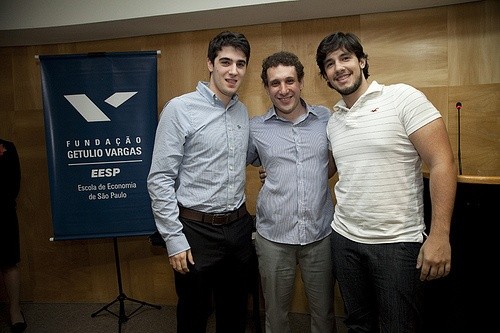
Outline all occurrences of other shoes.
[11,310,27,333]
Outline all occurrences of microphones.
[456,102,463,175]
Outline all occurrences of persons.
[259,32,458,333]
[147,29,266,332]
[249,51,335,333]
[0,138,28,333]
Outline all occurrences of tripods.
[91,236,161,333]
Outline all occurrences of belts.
[179,205,246,225]
